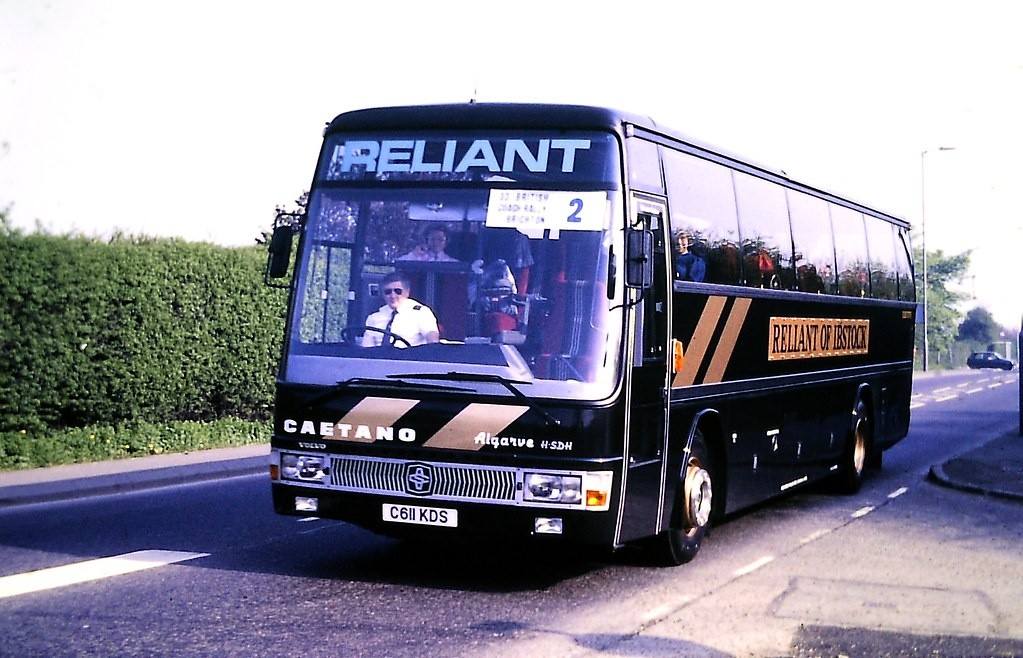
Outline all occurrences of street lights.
[919,145,957,372]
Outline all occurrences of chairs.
[686,237,914,302]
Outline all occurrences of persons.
[397,223,534,316]
[673,225,705,283]
[362,273,439,348]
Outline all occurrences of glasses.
[427,237,447,242]
[384,288,405,295]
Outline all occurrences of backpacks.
[481,272,519,316]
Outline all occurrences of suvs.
[967,351,1015,371]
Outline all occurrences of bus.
[263,101,917,572]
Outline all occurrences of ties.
[434,255,438,261]
[381,310,398,346]
